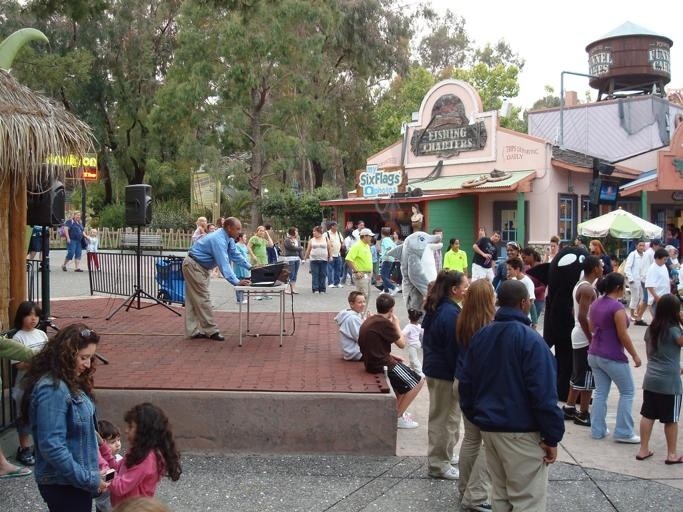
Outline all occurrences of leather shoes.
[190,333,209,339]
[209,332,225,341]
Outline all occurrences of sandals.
[633,320,649,326]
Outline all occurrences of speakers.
[124,183,152,226]
[27,176,65,228]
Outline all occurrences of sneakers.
[450,453,460,466]
[397,412,418,429]
[441,464,460,480]
[562,405,577,421]
[16,445,36,466]
[615,433,641,445]
[475,502,492,512]
[312,283,400,297]
[573,410,591,427]
[605,427,610,437]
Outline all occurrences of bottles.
[483,253,492,266]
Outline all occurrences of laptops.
[249,264,295,287]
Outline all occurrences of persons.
[302,219,681,511]
[94,403,183,512]
[84,229,101,271]
[25,226,51,272]
[0,336,40,479]
[95,420,124,512]
[182,217,303,340]
[61,210,86,272]
[10,301,48,465]
[17,322,112,512]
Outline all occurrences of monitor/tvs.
[590,178,619,207]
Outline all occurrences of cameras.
[101,468,115,482]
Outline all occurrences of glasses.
[80,328,96,338]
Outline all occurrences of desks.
[235,282,294,349]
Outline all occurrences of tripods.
[0,226,108,365]
[105,228,182,320]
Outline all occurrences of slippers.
[635,449,654,461]
[0,464,32,478]
[664,456,683,465]
[75,268,83,272]
[61,264,67,272]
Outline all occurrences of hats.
[360,228,376,237]
